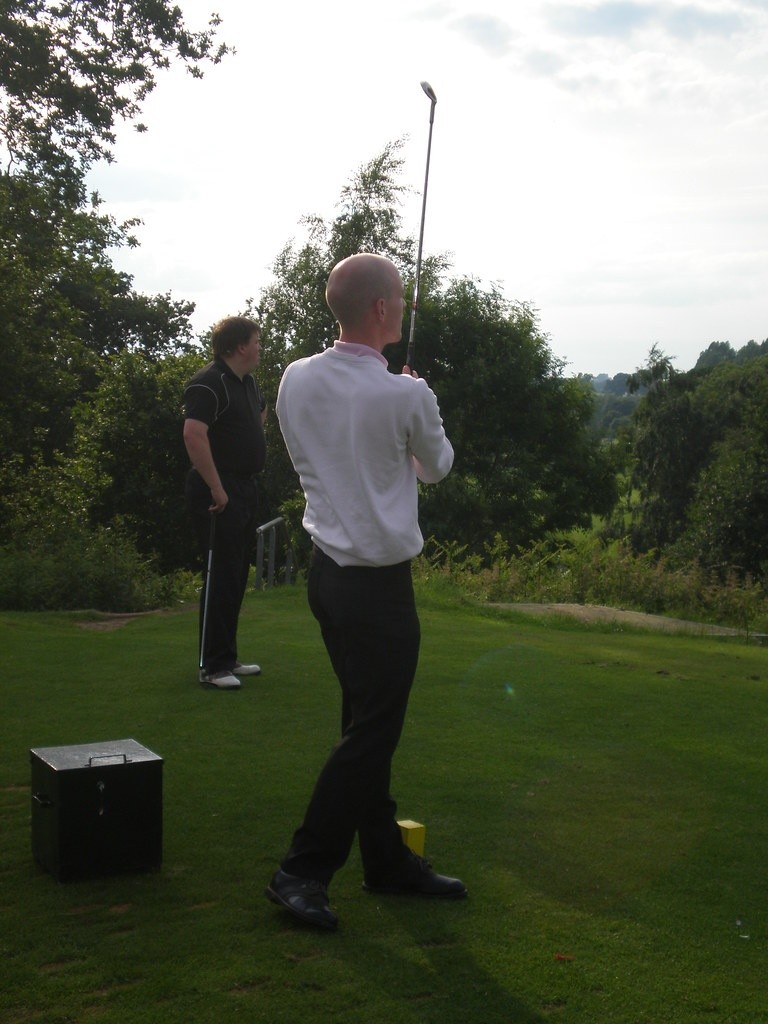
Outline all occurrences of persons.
[180,315,268,689]
[263,253,466,933]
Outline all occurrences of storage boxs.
[29,737,164,882]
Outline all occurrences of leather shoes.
[362,848,468,899]
[265,870,339,930]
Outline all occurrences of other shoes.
[233,661,260,676]
[199,670,240,690]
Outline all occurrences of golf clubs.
[407,81,437,368]
